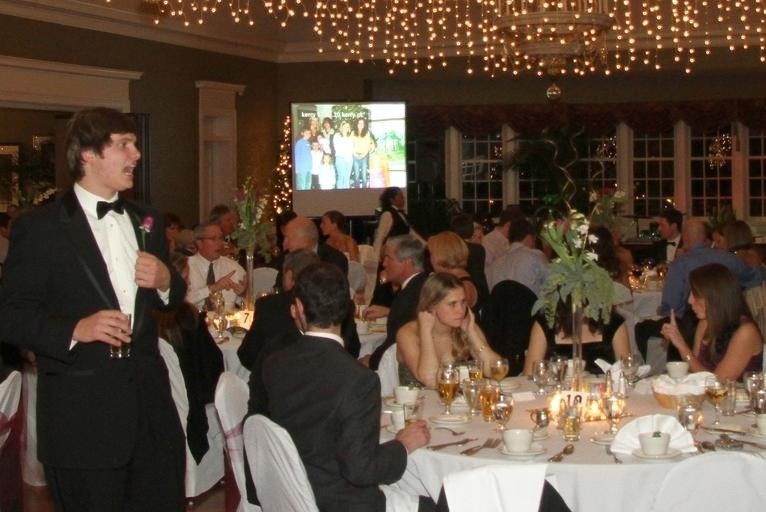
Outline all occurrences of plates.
[386,425,404,434]
[590,437,614,445]
[632,448,681,459]
[498,444,545,457]
[385,398,402,408]
[491,381,520,392]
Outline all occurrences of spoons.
[547,444,574,463]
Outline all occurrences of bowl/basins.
[653,388,706,411]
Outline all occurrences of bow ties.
[397,209,406,212]
[665,241,675,246]
[96,198,125,218]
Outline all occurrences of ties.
[205,264,216,310]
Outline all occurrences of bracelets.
[471,342,488,356]
[680,351,697,365]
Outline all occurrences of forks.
[460,437,499,456]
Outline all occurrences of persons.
[1,187,763,317]
[235,249,362,370]
[294,116,391,190]
[395,271,504,389]
[258,260,432,511]
[658,262,764,385]
[148,253,222,467]
[522,285,632,378]
[0,105,186,512]
[358,232,429,371]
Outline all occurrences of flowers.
[224,175,278,264]
[0,139,64,209]
[494,114,633,330]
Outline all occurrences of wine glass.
[628,257,667,293]
[532,352,640,435]
[678,370,766,438]
[434,358,513,443]
[194,287,282,341]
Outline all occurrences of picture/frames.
[0,145,22,215]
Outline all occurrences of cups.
[391,386,418,430]
[355,321,369,335]
[503,428,533,453]
[562,416,581,442]
[666,362,689,378]
[109,313,133,358]
[639,432,671,456]
